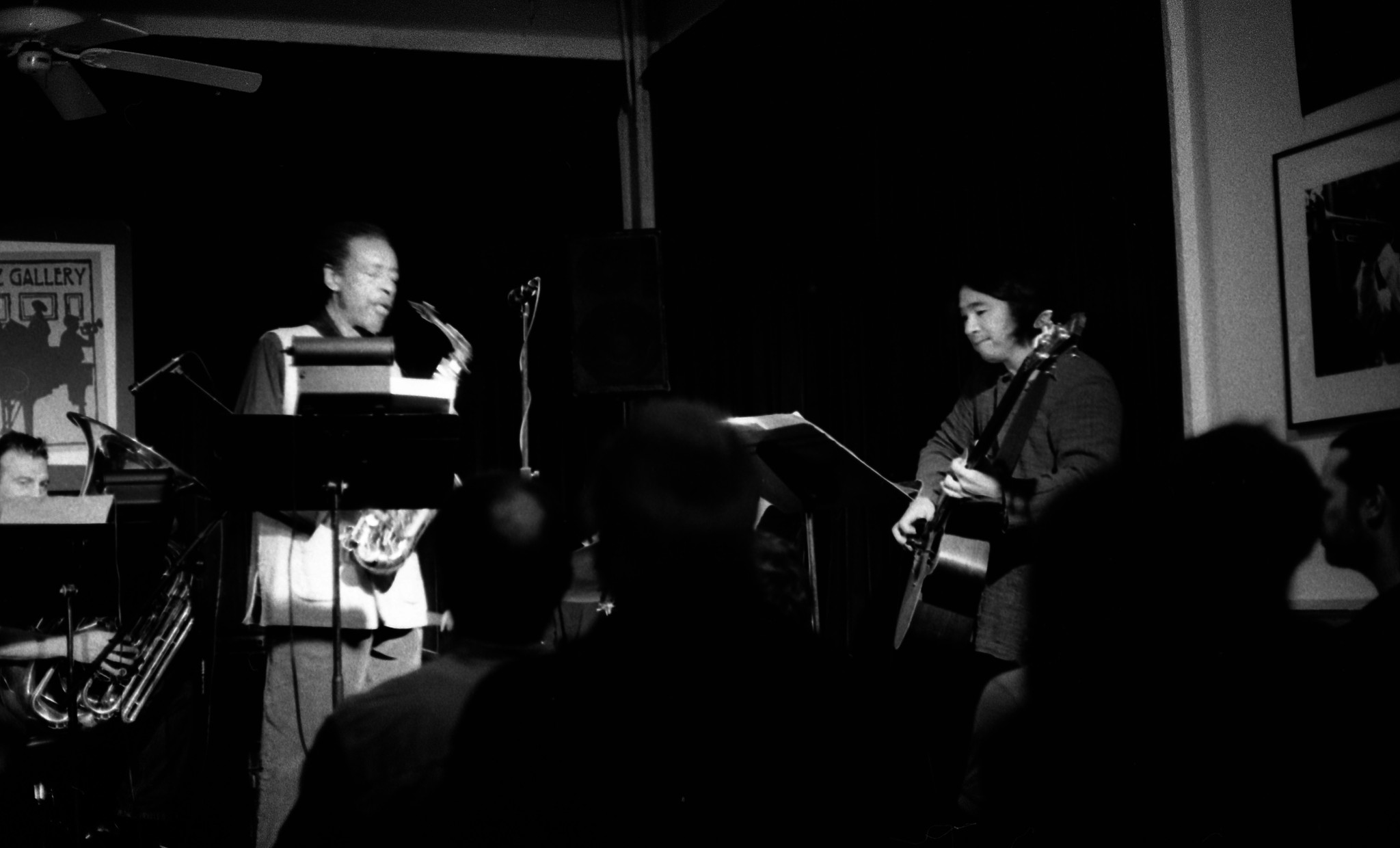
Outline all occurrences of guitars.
[888,308,1087,654]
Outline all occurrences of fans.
[0,0,263,123]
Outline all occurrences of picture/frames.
[1271,108,1400,438]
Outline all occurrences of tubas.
[18,410,211,733]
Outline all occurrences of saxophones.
[337,298,473,578]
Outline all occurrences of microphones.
[129,352,185,396]
[507,279,537,304]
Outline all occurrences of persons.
[272,408,1399,848]
[891,268,1121,661]
[237,225,457,848]
[0,429,140,675]
